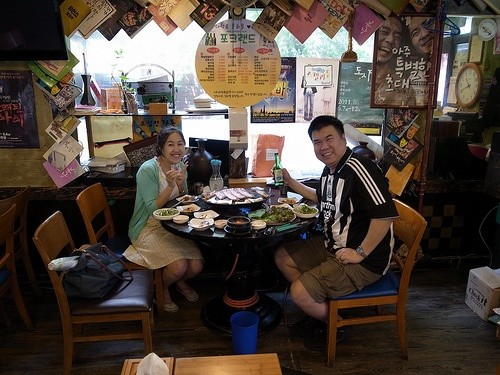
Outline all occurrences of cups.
[230,311,259,355]
[188,182,203,195]
[280,185,288,195]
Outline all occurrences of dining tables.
[159,189,322,334]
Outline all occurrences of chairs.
[0,183,428,375]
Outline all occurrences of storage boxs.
[464,266,500,322]
[149,103,168,114]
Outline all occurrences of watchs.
[356,245,368,260]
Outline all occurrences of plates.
[278,198,297,205]
[252,203,296,226]
[224,225,253,236]
[194,210,219,219]
[176,195,199,204]
[176,204,201,214]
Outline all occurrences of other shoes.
[304,328,344,350]
[290,316,326,335]
[176,286,200,301]
[153,297,178,311]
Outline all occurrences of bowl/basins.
[215,220,227,228]
[188,218,214,231]
[251,221,267,230]
[227,216,251,232]
[292,207,319,219]
[173,215,189,224]
[153,208,181,221]
[250,186,271,196]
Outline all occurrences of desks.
[121,353,282,375]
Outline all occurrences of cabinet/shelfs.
[68,108,230,200]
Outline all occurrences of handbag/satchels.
[58,242,133,307]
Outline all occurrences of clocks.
[478,18,498,42]
[455,64,482,109]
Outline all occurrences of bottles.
[273,152,284,186]
[188,138,215,187]
[80,75,96,106]
[209,160,223,192]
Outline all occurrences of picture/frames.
[371,12,437,109]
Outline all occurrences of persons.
[126,125,205,313]
[301,66,317,121]
[375,14,434,111]
[271,115,400,350]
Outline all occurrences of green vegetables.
[157,208,177,216]
[261,204,317,223]
